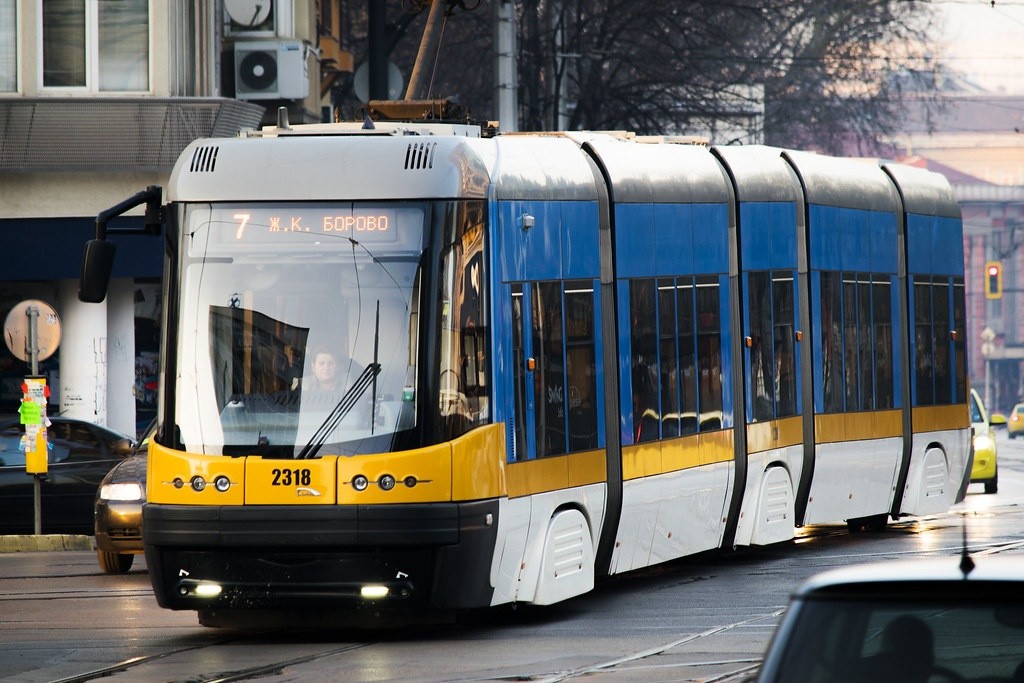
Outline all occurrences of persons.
[292,346,344,395]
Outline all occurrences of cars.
[756,551,1024,683]
[967,388,1008,495]
[0,415,138,536]
[93,412,159,575]
[1007,403,1024,438]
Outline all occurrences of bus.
[79,99,975,631]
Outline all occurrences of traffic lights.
[984,261,1003,301]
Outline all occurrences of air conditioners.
[234,40,309,99]
